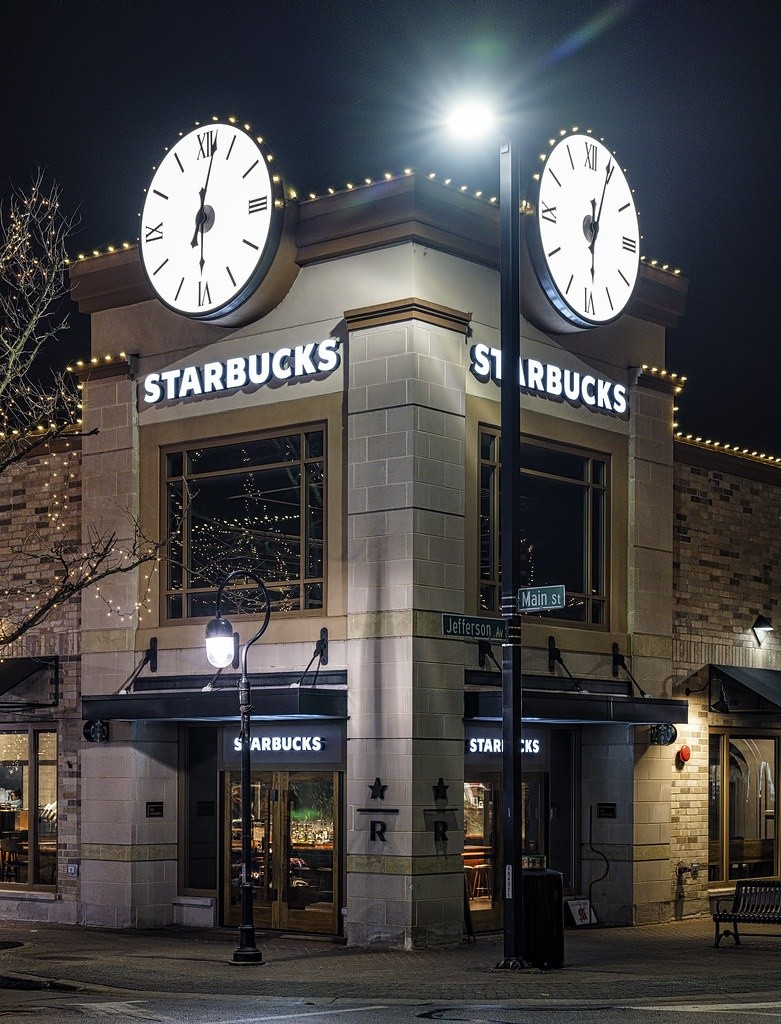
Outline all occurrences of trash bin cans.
[522,868,566,966]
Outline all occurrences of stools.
[294,867,310,886]
[318,868,333,894]
[464,865,473,898]
[471,864,492,899]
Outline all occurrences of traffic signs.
[501,583,567,614]
[441,613,521,647]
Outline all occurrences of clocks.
[539,134,639,323]
[140,122,272,317]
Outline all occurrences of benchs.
[713,881,781,948]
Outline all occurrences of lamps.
[684,678,730,715]
[751,614,775,647]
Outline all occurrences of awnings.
[465,649,688,723]
[707,664,781,710]
[82,652,346,720]
[0,656,59,705]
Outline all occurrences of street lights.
[435,89,521,974]
[203,567,270,965]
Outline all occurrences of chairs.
[1,839,27,882]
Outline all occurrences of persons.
[5,790,22,807]
